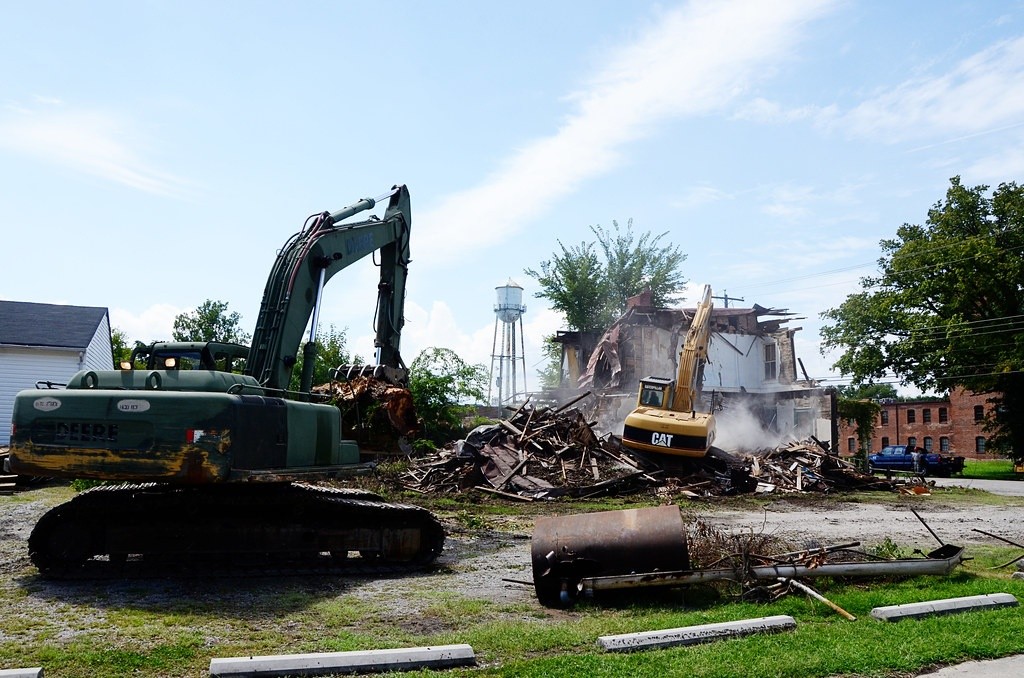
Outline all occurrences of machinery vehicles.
[10,182,444,586]
[623,284,746,490]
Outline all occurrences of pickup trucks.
[869,445,964,477]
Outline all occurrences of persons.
[910,446,924,473]
[648,390,660,406]
